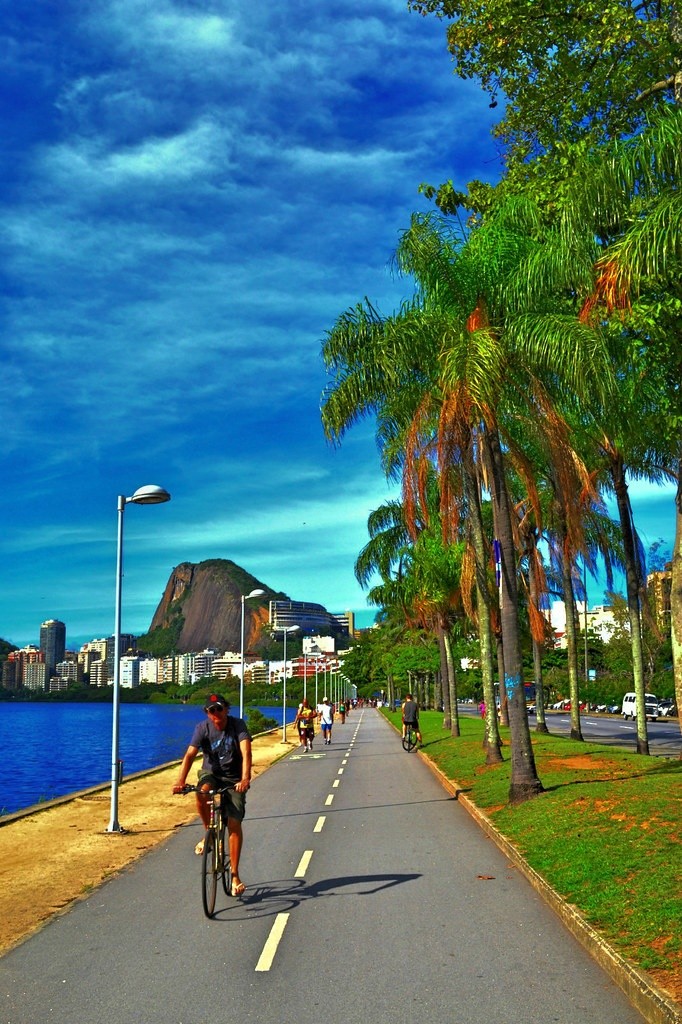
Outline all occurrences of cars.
[658,700,677,717]
[553,699,622,714]
[386,699,402,708]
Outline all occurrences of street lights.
[283,626,301,743]
[239,589,266,719]
[109,482,172,835]
[304,643,358,714]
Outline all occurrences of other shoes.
[402,737,405,741]
[420,745,424,747]
[303,748,308,753]
[327,740,331,745]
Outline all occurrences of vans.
[621,692,658,722]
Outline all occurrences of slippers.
[231,882,243,896]
[194,842,204,854]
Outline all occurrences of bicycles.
[173,785,250,918]
[402,723,417,753]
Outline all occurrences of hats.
[323,697,327,701]
[205,694,224,710]
[303,699,307,707]
[407,694,411,698]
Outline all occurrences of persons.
[172,695,253,896]
[479,700,485,719]
[293,702,306,747]
[329,697,377,716]
[401,694,424,748]
[318,696,334,745]
[339,699,347,724]
[298,699,316,753]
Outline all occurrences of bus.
[494,680,536,715]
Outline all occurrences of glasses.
[209,706,223,713]
